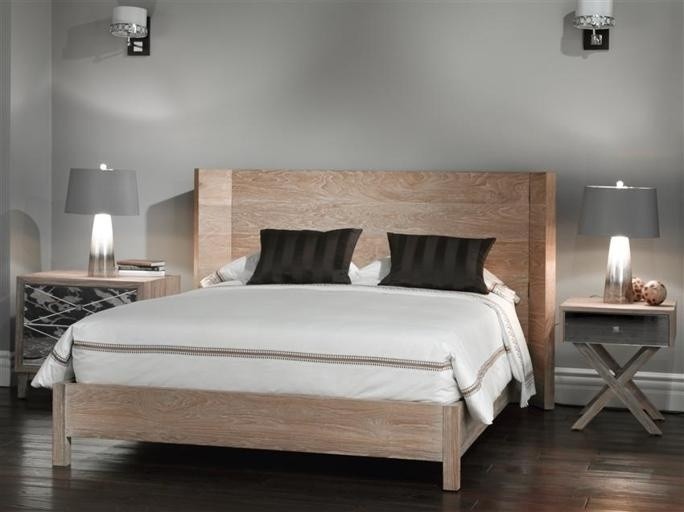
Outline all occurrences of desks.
[561,299,674,432]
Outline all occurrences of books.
[118,264,166,271]
[118,270,167,277]
[117,258,167,267]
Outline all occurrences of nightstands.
[14,273,182,399]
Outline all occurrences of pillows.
[247,224,498,293]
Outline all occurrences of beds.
[30,168,557,486]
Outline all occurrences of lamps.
[576,183,660,303]
[110,5,150,56]
[65,166,143,276]
[574,1,614,51]
